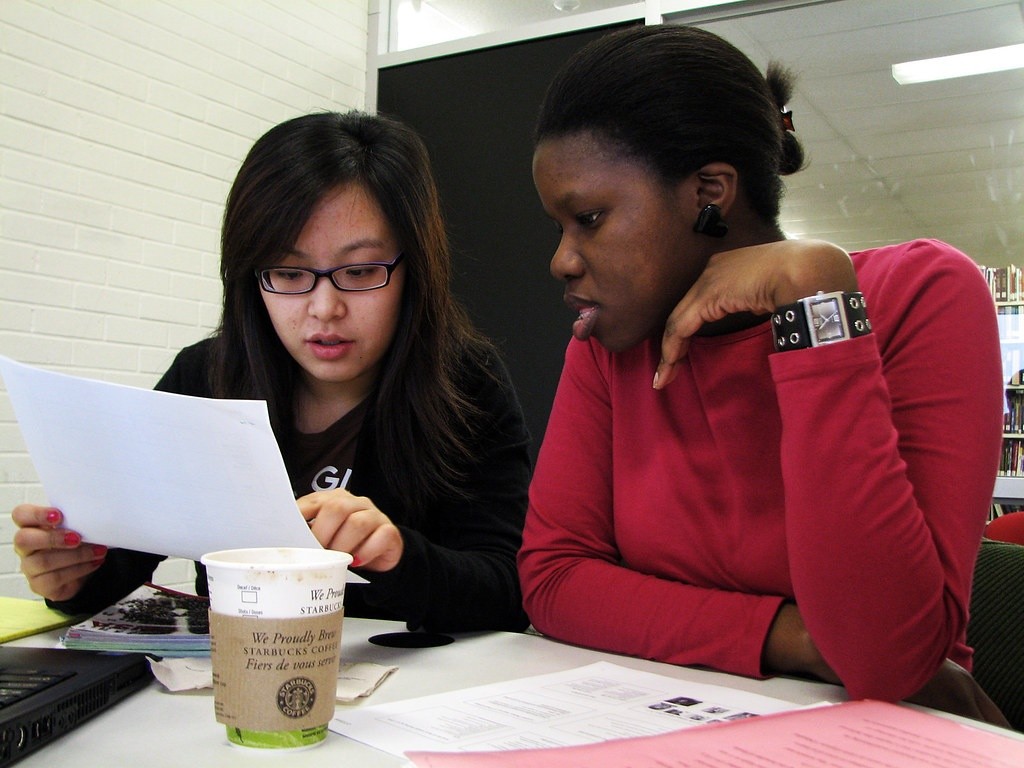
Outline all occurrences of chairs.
[965,537,1024,735]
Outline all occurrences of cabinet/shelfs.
[986,301,1024,525]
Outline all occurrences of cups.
[200,546,353,751]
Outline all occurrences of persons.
[11,109,536,635]
[517,25,1012,730]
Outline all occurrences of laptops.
[0,645,159,767]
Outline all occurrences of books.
[63,581,212,658]
[998,393,1024,477]
[977,264,1024,315]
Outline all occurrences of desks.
[0,615,1024,768]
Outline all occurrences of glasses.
[254,248,406,296]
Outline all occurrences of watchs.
[770,290,872,353]
